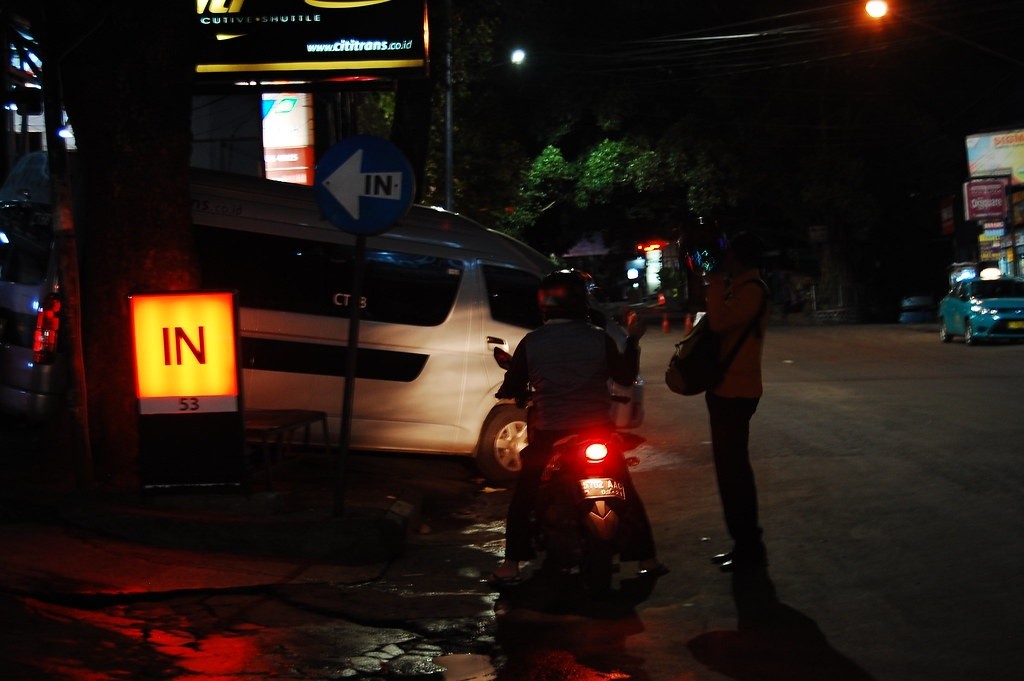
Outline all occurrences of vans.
[0,149,647,485]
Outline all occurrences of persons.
[484,268,670,579]
[693,220,768,572]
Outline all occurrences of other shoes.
[711,549,770,571]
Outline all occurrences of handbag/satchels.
[664,280,769,394]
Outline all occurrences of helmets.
[537,268,608,329]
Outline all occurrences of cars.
[936,275,1024,346]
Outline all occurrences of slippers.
[484,568,522,585]
[638,555,673,574]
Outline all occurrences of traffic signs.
[313,134,415,236]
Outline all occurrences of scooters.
[494,313,642,598]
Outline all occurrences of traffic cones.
[659,312,672,334]
[682,313,693,337]
